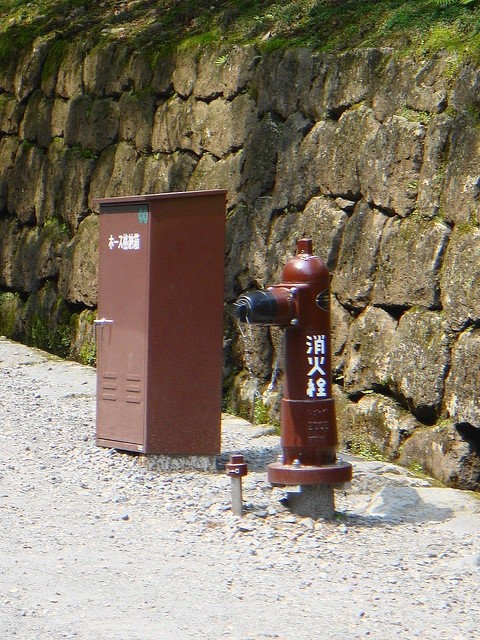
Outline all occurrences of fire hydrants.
[233,238,352,520]
